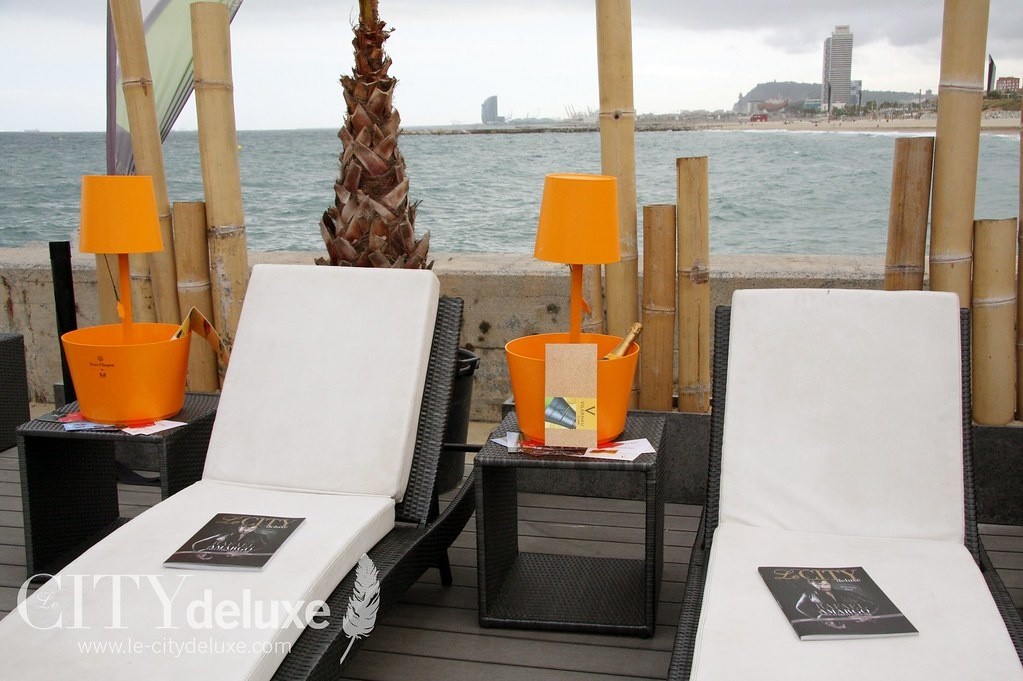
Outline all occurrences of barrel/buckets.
[435,348,481,496]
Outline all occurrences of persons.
[793,577,881,629]
[193,517,270,562]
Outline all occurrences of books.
[163,512,304,571]
[757,567,921,638]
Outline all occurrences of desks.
[472,413,663,634]
[17,394,218,581]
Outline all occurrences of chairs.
[667,287,1023,681]
[0,266,486,681]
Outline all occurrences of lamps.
[505,174,640,443]
[62,175,194,424]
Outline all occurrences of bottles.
[599,322,643,360]
